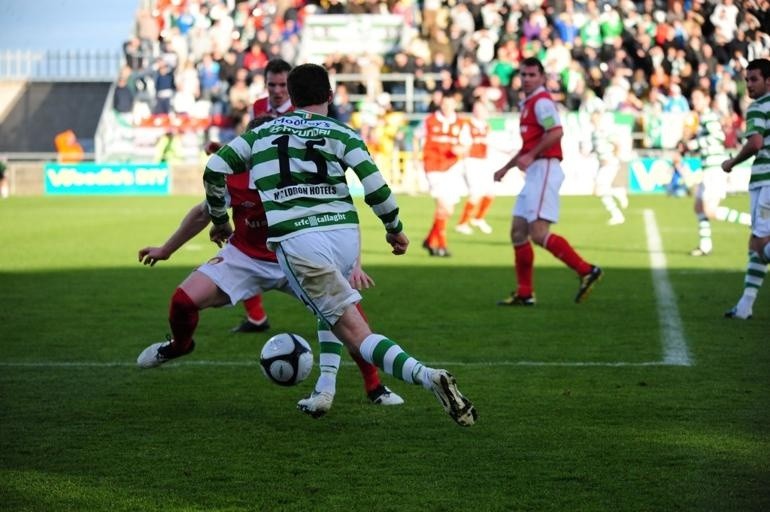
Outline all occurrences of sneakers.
[366,384,405,405]
[455,218,492,235]
[607,186,629,225]
[230,315,270,333]
[429,368,478,426]
[575,264,605,304]
[496,291,537,305]
[724,306,752,319]
[689,246,713,257]
[296,385,334,418]
[423,240,450,256]
[136,333,195,369]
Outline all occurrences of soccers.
[260,333,314,385]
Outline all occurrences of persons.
[114,1,769,151]
[493,58,603,306]
[585,107,629,227]
[414,93,501,256]
[203,65,478,426]
[722,58,770,321]
[672,87,752,257]
[137,116,404,407]
[228,58,292,333]
